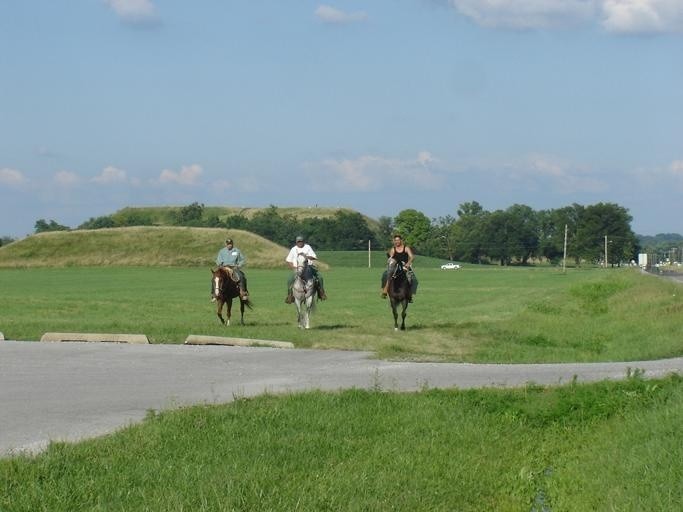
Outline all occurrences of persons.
[284,236,328,304]
[211,238,248,304]
[381,235,415,304]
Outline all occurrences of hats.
[296,236,304,242]
[226,238,232,243]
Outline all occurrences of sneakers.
[410,297,414,302]
[382,291,387,296]
[318,289,327,299]
[285,294,294,304]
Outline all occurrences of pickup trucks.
[440,263,459,270]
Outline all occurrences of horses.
[211,265,254,327]
[292,250,317,330]
[380,252,410,331]
